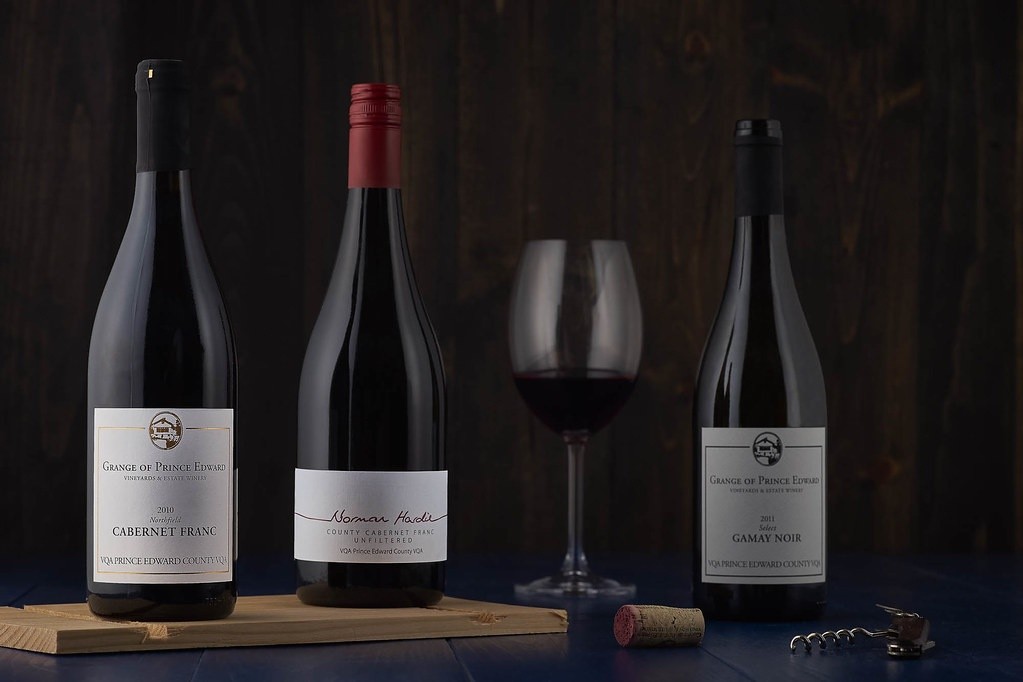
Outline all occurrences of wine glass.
[507,240,643,598]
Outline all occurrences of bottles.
[85,59,239,623]
[692,119,826,625]
[297,82,447,609]
